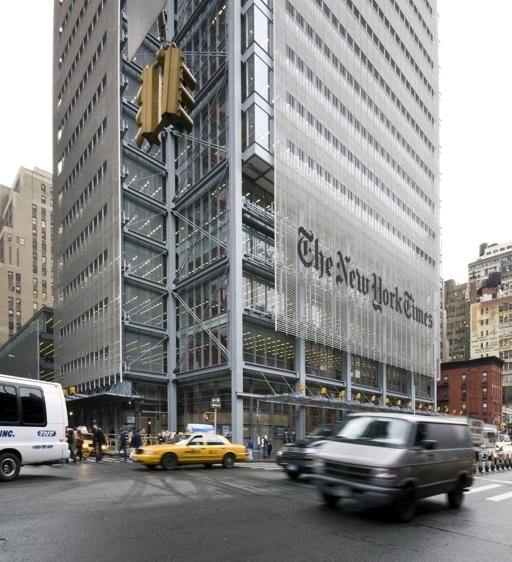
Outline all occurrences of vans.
[276,412,477,523]
[0,374,71,482]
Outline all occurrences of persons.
[67,426,143,465]
[246,436,272,460]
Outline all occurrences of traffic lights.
[134,43,197,149]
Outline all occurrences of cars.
[79,433,109,461]
[129,432,248,470]
